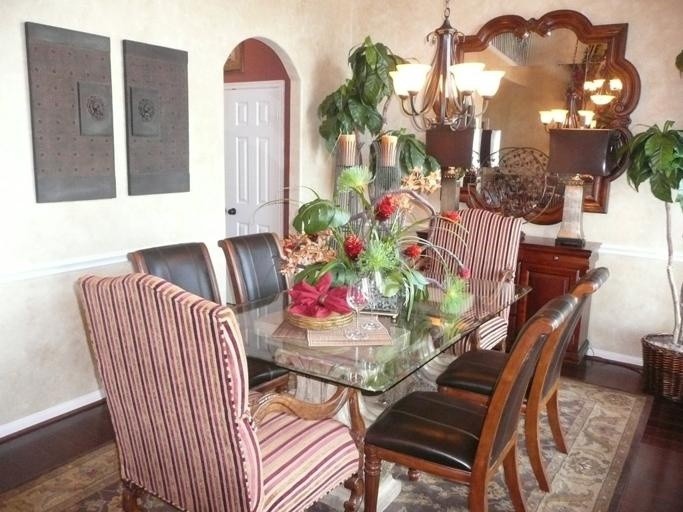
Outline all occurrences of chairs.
[435,265,608,493]
[221,230,297,308]
[361,293,581,506]
[77,272,370,512]
[125,241,290,399]
[416,207,525,361]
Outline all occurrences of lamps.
[439,69,505,119]
[389,1,483,127]
[539,37,623,134]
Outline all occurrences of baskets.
[641,331,683,405]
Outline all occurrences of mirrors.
[433,8,640,226]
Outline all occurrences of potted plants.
[607,120,682,403]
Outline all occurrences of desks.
[194,262,533,506]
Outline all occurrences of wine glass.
[344,270,385,342]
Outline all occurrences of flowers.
[249,165,472,310]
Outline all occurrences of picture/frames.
[223,41,244,72]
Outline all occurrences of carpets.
[0,373,655,511]
